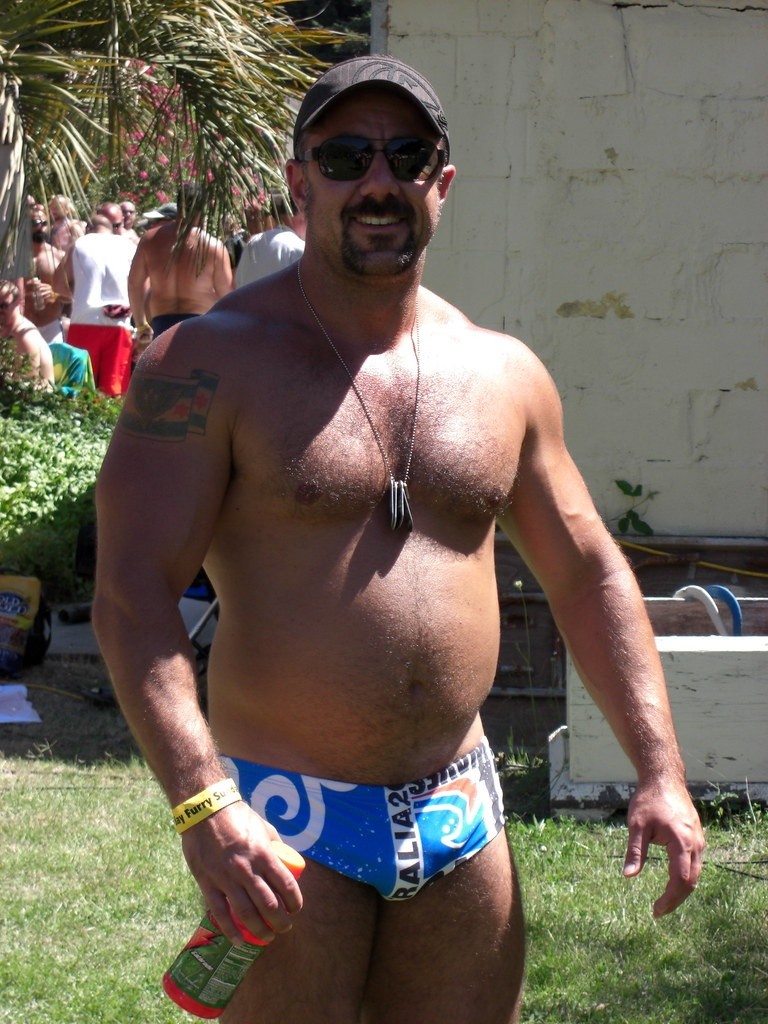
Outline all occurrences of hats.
[293,55,452,163]
[142,202,178,219]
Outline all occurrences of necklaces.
[297,260,420,532]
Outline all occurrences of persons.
[92,56,704,1024]
[0,187,308,396]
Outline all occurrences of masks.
[33,231,47,243]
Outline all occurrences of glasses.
[0,301,14,310]
[296,135,445,182]
[113,219,123,228]
[29,203,38,210]
[122,210,136,215]
[32,219,48,227]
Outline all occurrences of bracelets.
[172,777,242,835]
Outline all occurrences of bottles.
[161,842,307,1022]
